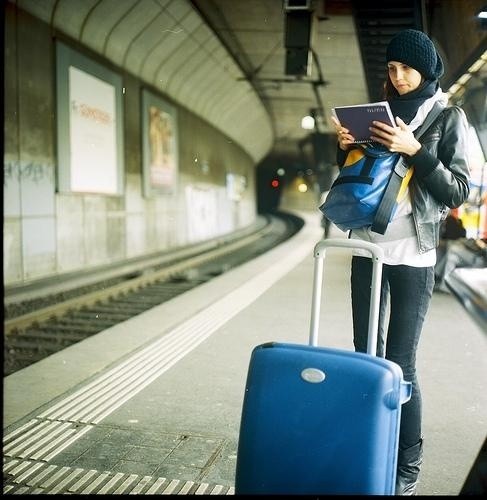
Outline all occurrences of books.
[330,101,396,144]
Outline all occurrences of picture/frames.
[52,32,180,202]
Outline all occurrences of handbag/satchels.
[319,133,411,235]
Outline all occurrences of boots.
[396,437,424,496]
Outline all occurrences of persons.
[430,235,486,294]
[319,30,481,494]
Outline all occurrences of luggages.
[235,238,412,495]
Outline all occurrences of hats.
[386,28,437,79]
